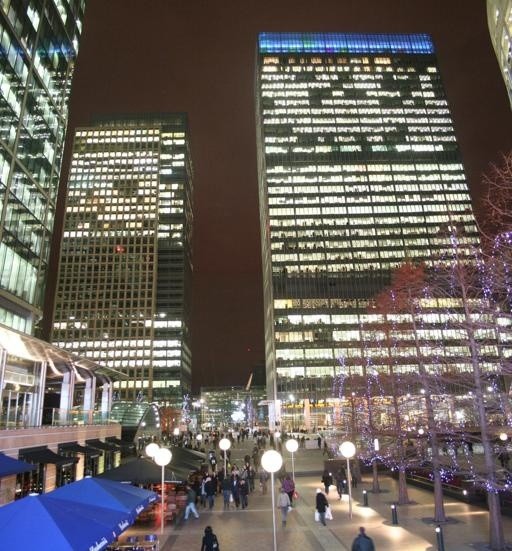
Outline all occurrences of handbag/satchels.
[212,541,217,551]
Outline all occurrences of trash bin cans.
[209,451,215,461]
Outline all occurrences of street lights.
[144,436,358,551]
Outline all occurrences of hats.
[316,488,321,493]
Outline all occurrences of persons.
[202,526,220,551]
[352,527,375,551]
[403,439,413,456]
[138,419,359,530]
[498,445,510,472]
[466,439,473,457]
